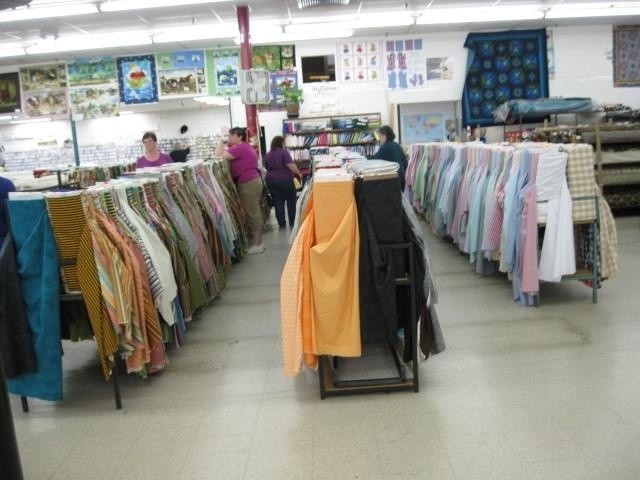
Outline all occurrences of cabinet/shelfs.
[281,112,382,176]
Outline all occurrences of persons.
[213,125,266,255]
[260,134,304,228]
[167,143,196,162]
[363,125,410,193]
[132,131,174,169]
